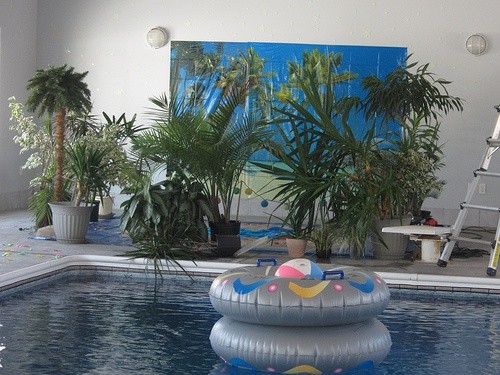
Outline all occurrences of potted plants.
[339,113,448,262]
[263,201,312,258]
[127,83,276,256]
[47,139,124,244]
[88,112,149,217]
[307,227,334,258]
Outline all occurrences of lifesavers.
[208,317,393,375]
[208,262,391,328]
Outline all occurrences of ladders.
[437,105,500,276]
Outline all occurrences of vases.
[83,200,101,222]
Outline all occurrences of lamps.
[465,33,488,56]
[146,28,168,49]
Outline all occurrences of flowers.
[7,97,124,196]
